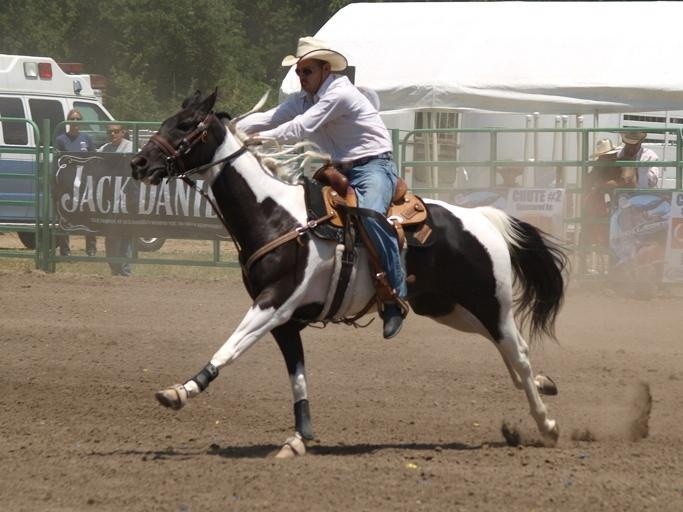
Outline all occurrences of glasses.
[106,128,122,134]
[68,117,82,120]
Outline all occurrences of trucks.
[0,53,169,250]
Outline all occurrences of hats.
[281,36,348,72]
[496,157,526,173]
[590,137,622,158]
[618,132,647,144]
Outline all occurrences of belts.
[353,155,377,166]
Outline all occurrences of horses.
[128,88,567,461]
[580,165,639,273]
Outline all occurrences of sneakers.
[383,302,402,339]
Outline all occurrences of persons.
[97,125,142,278]
[234,36,408,339]
[589,127,660,188]
[55,109,96,256]
